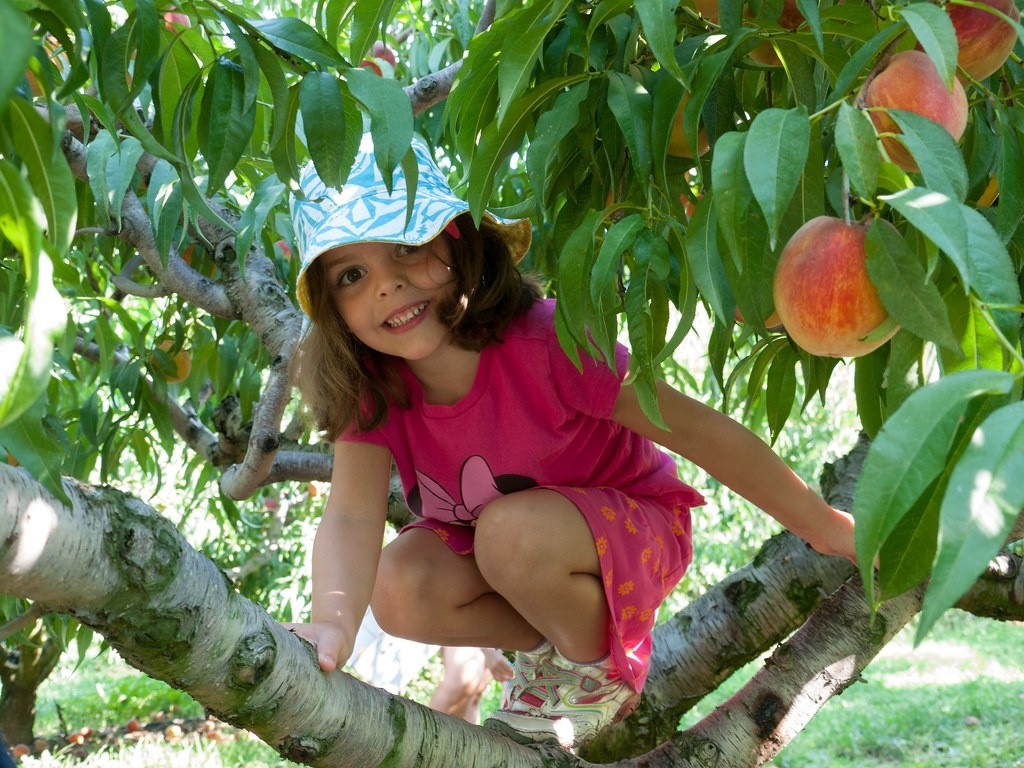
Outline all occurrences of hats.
[289,138,532,320]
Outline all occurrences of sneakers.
[483,649,641,749]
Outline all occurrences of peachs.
[0,0,1015,384]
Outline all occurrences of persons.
[280,133,881,746]
[431,643,517,728]
